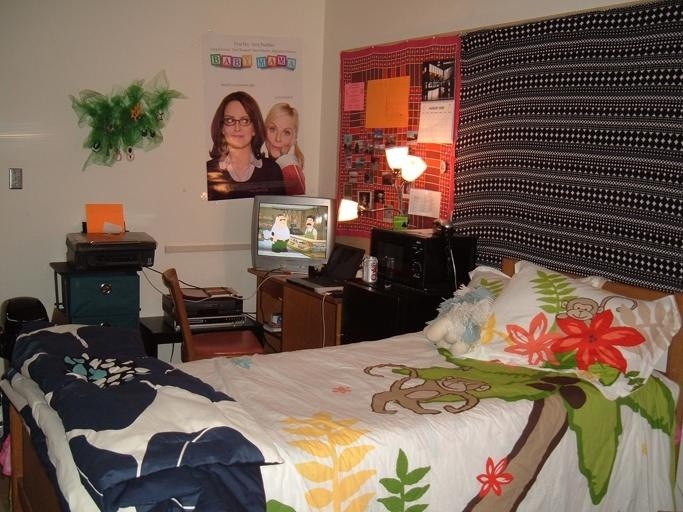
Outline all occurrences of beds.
[0,251,683,512]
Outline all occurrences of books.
[263,320,281,328]
[262,323,282,333]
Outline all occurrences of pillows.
[422,260,681,401]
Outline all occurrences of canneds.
[362,257,378,283]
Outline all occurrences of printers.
[66,232,157,271]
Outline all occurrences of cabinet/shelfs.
[48,260,141,329]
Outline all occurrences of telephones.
[433,218,453,232]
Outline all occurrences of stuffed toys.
[423,283,496,358]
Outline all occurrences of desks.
[245,266,398,352]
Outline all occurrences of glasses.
[219,116,254,126]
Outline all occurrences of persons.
[206,91,286,199]
[263,101,307,195]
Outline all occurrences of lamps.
[335,144,428,224]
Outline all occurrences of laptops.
[309,243,365,287]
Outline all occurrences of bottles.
[364,259,378,284]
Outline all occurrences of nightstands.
[339,276,453,345]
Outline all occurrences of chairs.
[159,266,263,363]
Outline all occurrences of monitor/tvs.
[250,195,336,272]
